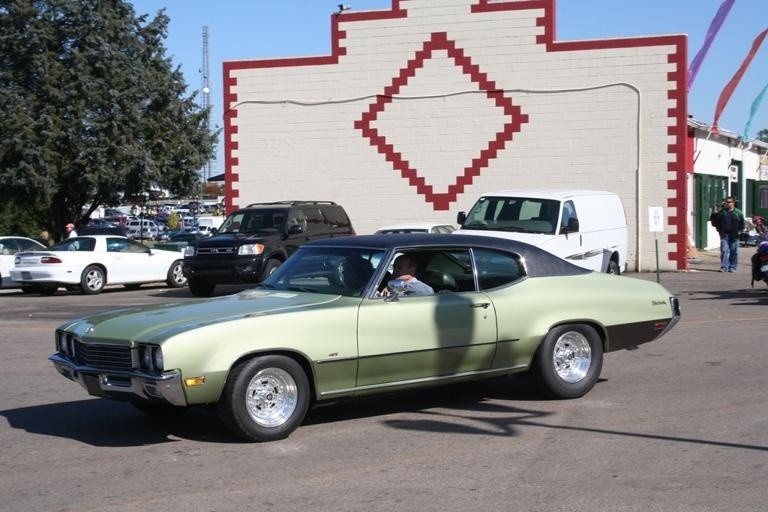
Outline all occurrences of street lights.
[202,87,211,183]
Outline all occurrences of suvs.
[177,197,359,298]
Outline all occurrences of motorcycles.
[744,231,767,288]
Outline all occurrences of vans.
[449,188,629,277]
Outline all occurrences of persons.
[380,254,434,299]
[711,197,745,273]
[39,230,50,247]
[65,223,80,251]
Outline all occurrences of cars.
[0,235,53,294]
[10,233,193,295]
[47,232,681,442]
[80,201,226,241]
[363,219,457,276]
